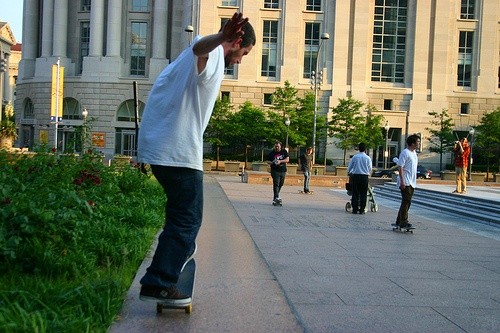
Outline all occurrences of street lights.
[467,127,476,181]
[311,33,330,164]
[82,107,88,155]
[285,117,291,149]
[184,24,194,47]
[385,120,389,169]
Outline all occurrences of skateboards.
[391,224,416,234]
[157,258,195,314]
[272,200,282,206]
[299,191,314,194]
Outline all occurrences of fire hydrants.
[313,168,320,175]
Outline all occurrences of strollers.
[345,187,378,214]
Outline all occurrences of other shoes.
[273,198,282,203]
[461,190,466,194]
[452,190,462,193]
[139,284,192,303]
[396,221,413,228]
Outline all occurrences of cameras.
[455,141,459,144]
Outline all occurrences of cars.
[371,163,433,180]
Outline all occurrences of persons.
[300,146,313,193]
[136,12,257,304]
[267,141,289,203]
[453,137,470,194]
[396,135,420,229]
[346,143,372,215]
